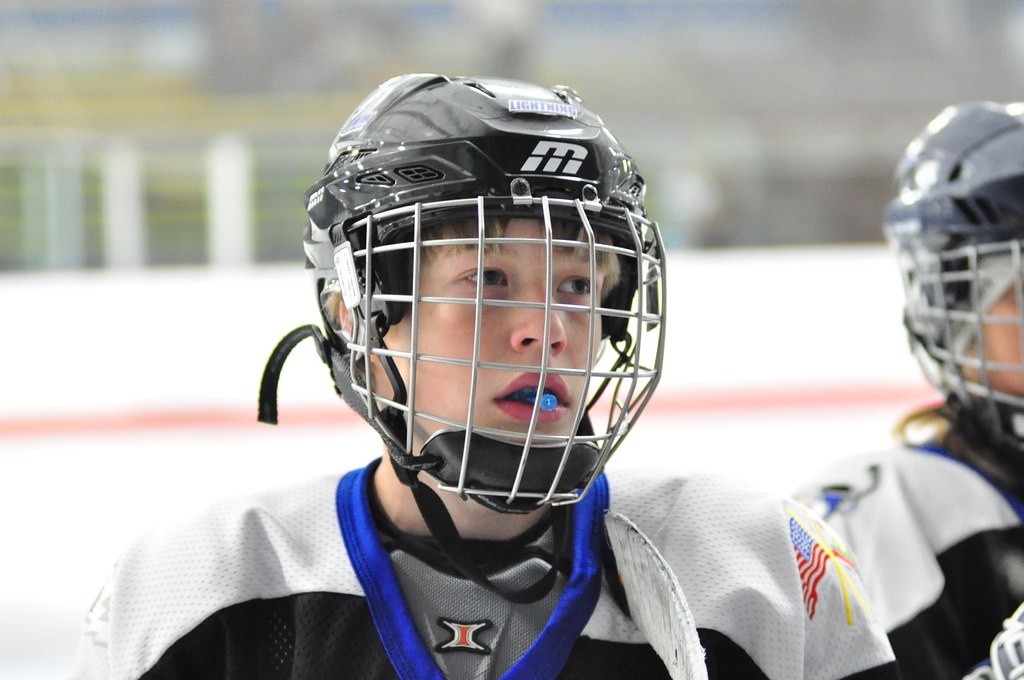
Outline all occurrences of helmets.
[887,99,1022,473]
[256,72,668,514]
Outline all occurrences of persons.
[74,73,900,680]
[816,101,1023,680]
